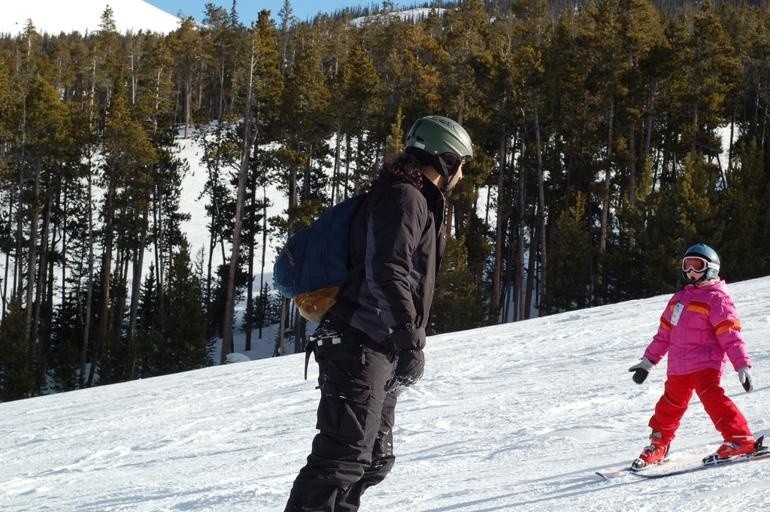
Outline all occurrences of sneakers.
[640,441,670,464]
[717,439,756,458]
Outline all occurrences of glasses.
[681,257,720,273]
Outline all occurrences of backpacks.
[274,191,378,324]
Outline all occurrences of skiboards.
[595,434,770,483]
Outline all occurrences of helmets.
[681,243,720,280]
[405,116,473,161]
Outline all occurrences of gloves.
[386,327,426,392]
[628,358,655,385]
[737,366,753,393]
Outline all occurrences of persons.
[628,244,757,471]
[285,114,478,512]
